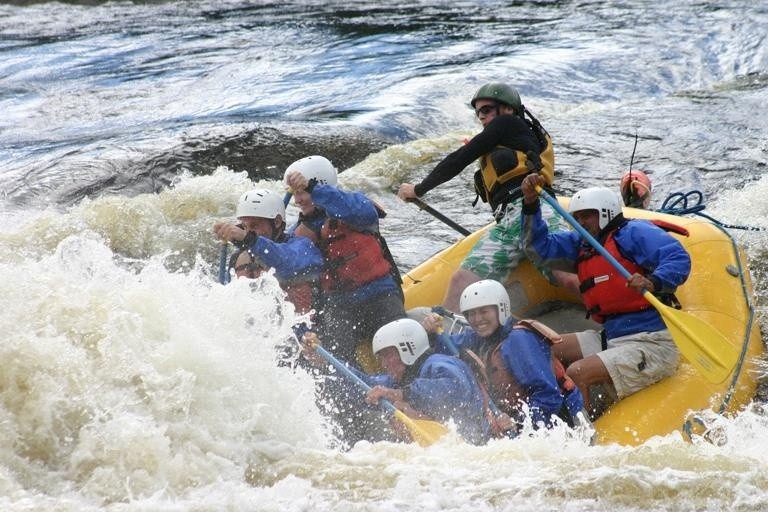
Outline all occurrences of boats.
[283,191,761,498]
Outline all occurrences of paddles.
[306,337,448,447]
[535,185,741,384]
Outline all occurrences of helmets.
[234,250,267,269]
[372,318,430,365]
[236,188,285,224]
[470,82,521,112]
[459,279,510,327]
[567,187,623,231]
[283,155,337,192]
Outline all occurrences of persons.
[396,81,556,318]
[518,172,691,426]
[213,187,323,370]
[301,317,491,451]
[285,155,406,392]
[422,279,586,445]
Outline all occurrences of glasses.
[475,104,501,116]
